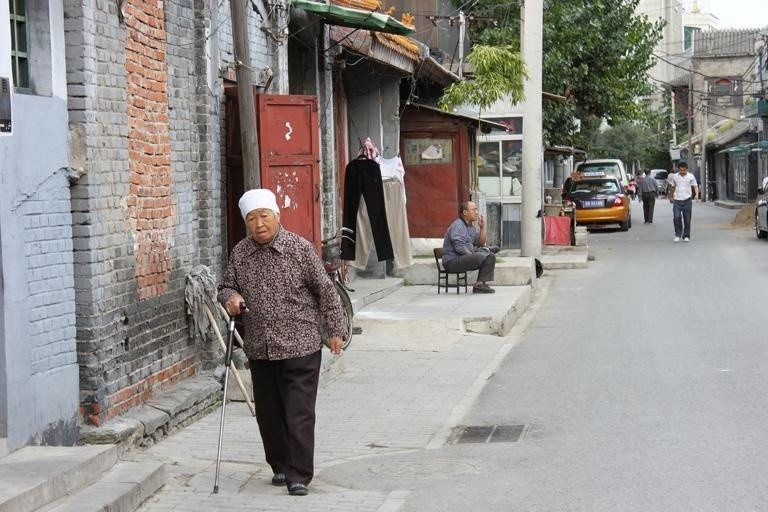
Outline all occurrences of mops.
[184,265,256,418]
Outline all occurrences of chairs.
[434,248,467,295]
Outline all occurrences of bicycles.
[308,224,357,349]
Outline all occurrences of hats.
[238,189,280,225]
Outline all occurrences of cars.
[626,172,638,200]
[561,171,631,233]
[754,176,768,238]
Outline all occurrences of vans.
[645,169,670,193]
[574,159,628,195]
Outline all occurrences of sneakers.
[272,473,286,486]
[473,284,495,293]
[287,480,308,495]
[673,237,679,242]
[683,237,689,242]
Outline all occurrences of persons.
[628,176,637,201]
[442,201,500,294]
[639,168,658,224]
[670,162,698,242]
[636,173,643,201]
[217,188,346,495]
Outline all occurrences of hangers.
[356,145,369,160]
